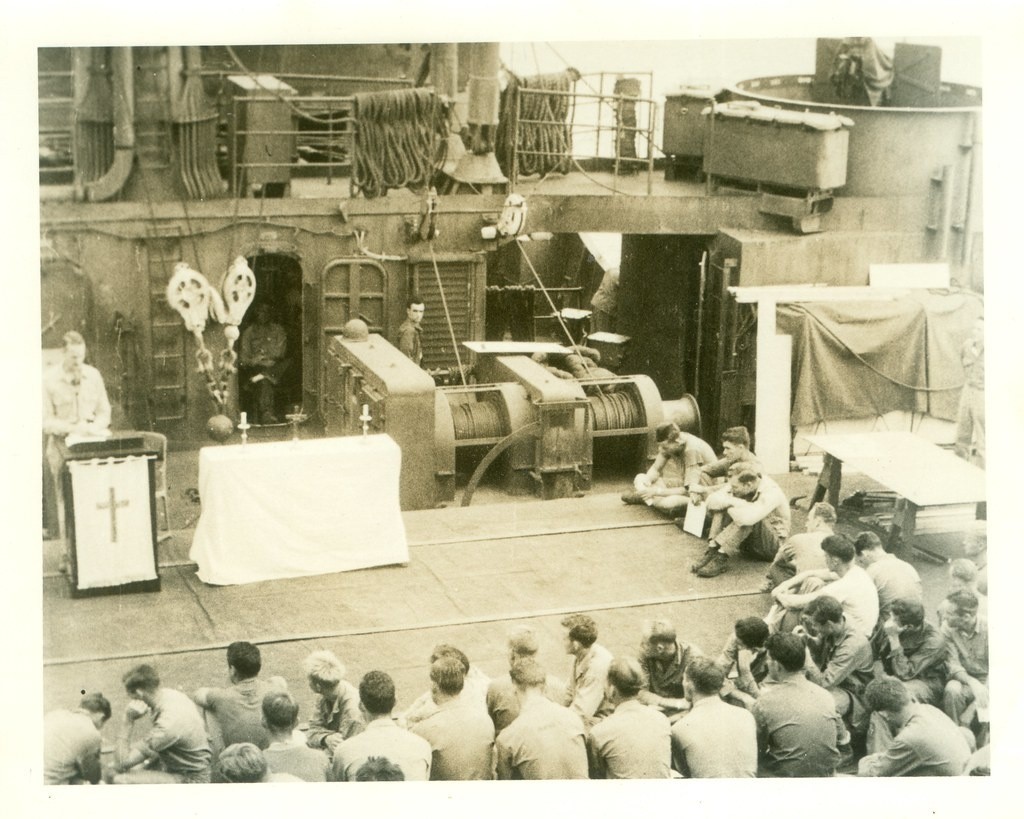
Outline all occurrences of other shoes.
[619,491,644,506]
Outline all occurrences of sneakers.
[690,544,734,578]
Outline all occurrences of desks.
[803,432,987,560]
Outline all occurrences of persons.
[751,633,853,778]
[857,679,971,778]
[588,656,671,779]
[621,423,717,516]
[397,293,424,369]
[332,671,431,782]
[690,424,791,578]
[45,595,874,785]
[769,501,838,585]
[869,596,946,704]
[942,560,991,749]
[854,532,923,618]
[42,330,112,572]
[773,533,879,639]
[589,268,622,337]
[494,658,588,781]
[406,657,496,782]
[670,656,758,779]
[239,297,289,423]
